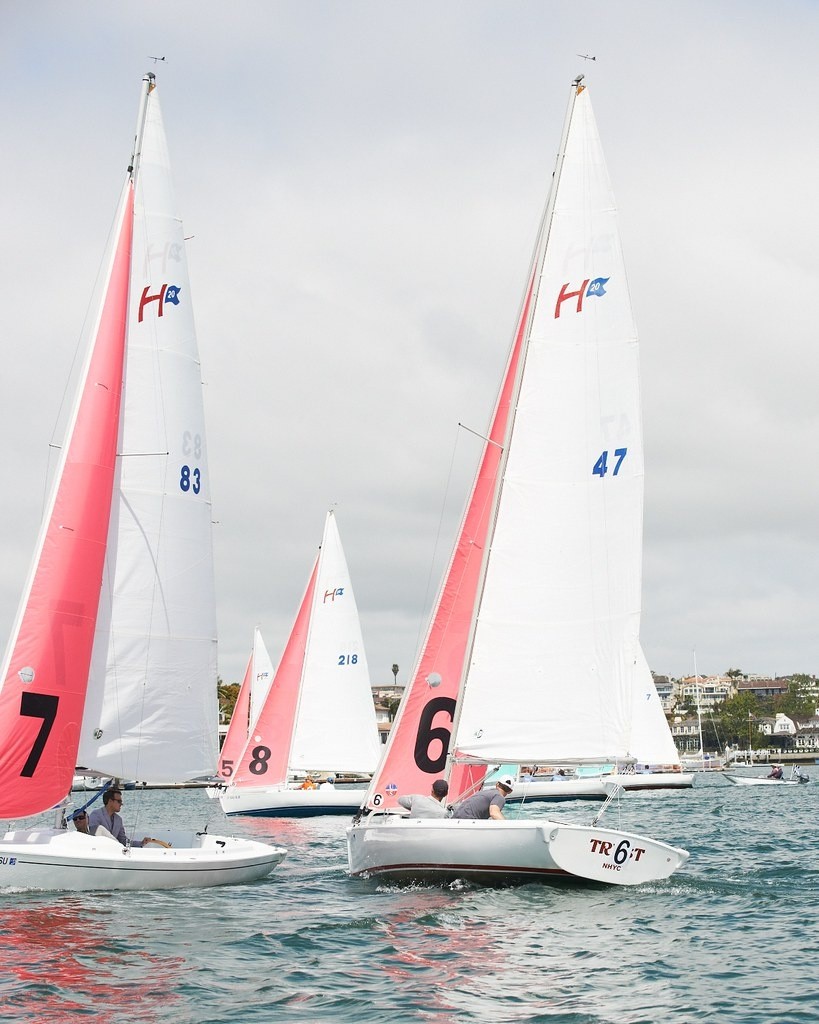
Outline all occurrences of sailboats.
[650,648,782,772]
[2,43,293,895]
[341,38,693,888]
[68,499,693,818]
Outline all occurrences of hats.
[328,777,333,783]
[432,780,448,796]
[771,764,776,767]
[498,775,515,790]
[305,775,313,780]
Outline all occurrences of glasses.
[112,798,122,803]
[73,816,86,821]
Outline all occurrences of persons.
[399,779,452,819]
[72,809,90,834]
[293,775,316,790]
[549,770,569,782]
[320,776,335,790]
[768,763,784,779]
[523,771,537,782]
[88,790,151,848]
[641,765,652,774]
[449,774,515,820]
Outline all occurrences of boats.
[722,763,810,787]
